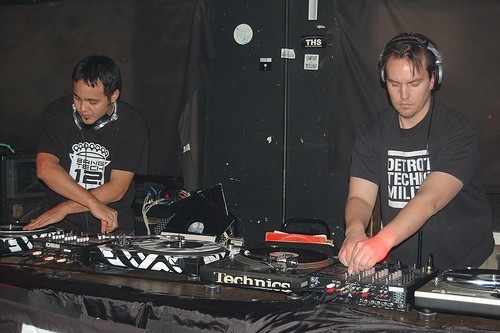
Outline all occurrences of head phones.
[379,33,443,94]
[73,101,118,130]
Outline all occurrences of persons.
[22,54,149,236]
[338,32,498,275]
